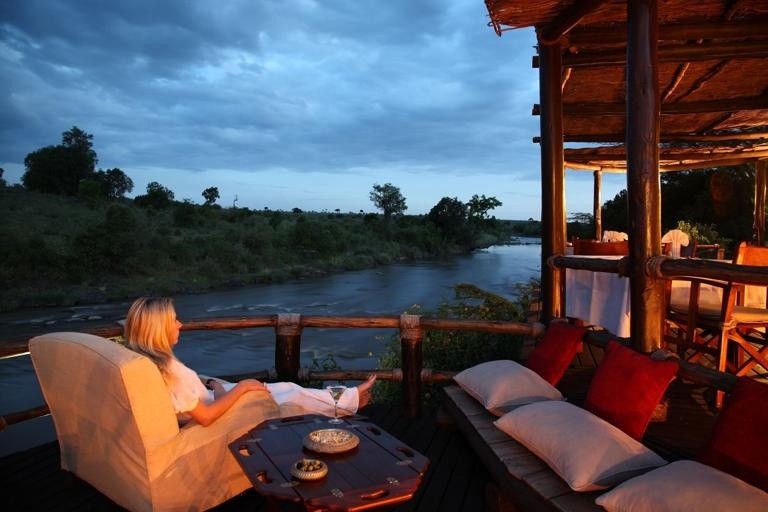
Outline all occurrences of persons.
[123,295,377,427]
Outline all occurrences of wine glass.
[326,386,346,424]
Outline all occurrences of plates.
[302,426,359,455]
[291,459,328,482]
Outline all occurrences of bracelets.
[205,378,216,391]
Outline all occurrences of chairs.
[673,241,768,408]
[26,332,281,511]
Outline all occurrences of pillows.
[528,324,587,382]
[596,459,768,512]
[708,375,767,491]
[452,357,561,417]
[493,400,668,492]
[585,341,680,440]
